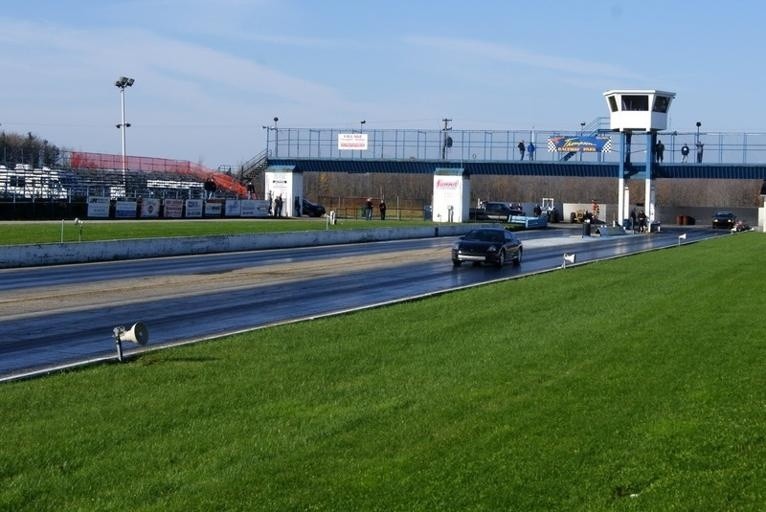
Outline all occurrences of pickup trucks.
[470,201,523,222]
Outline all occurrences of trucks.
[0,163,77,201]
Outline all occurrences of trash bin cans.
[582,222,591,238]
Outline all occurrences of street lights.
[272,114,281,159]
[695,119,705,164]
[113,72,135,198]
[358,119,367,157]
[578,121,589,161]
[442,126,453,160]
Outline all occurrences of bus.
[144,179,216,200]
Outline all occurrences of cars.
[711,211,739,229]
[451,227,526,269]
[300,196,328,220]
[423,202,432,220]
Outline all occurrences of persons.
[267,192,282,218]
[510,202,523,211]
[696,141,704,163]
[527,142,535,161]
[203,177,216,200]
[656,140,665,163]
[247,181,255,200]
[379,199,386,220]
[630,208,636,230]
[637,210,646,233]
[518,140,526,161]
[680,143,689,163]
[534,204,539,217]
[365,198,373,221]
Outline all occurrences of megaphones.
[565,254,576,263]
[679,233,687,240]
[120,321,148,345]
[74,217,83,225]
